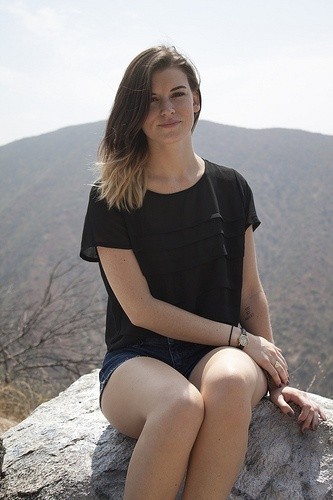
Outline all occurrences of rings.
[273,361,280,369]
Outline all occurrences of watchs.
[237,327,248,351]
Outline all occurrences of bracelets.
[228,325,233,347]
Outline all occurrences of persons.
[79,45,327,500]
